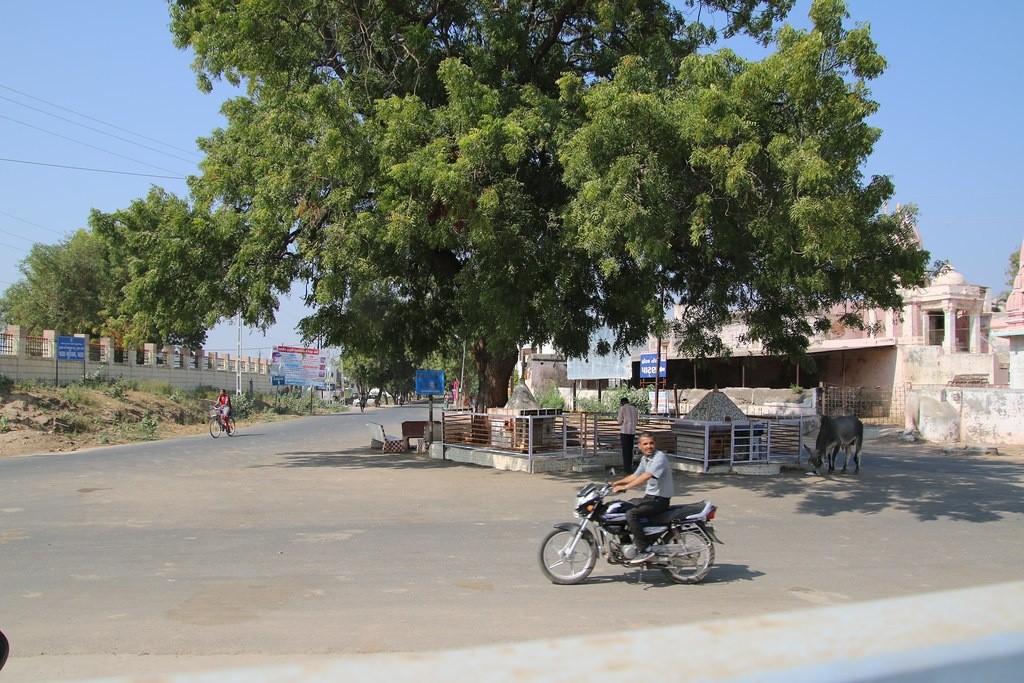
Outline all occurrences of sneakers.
[630,549,655,564]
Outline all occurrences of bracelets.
[623,485,629,491]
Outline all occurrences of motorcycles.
[538,467,723,586]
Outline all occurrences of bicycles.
[359,399,366,412]
[209,405,236,439]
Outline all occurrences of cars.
[340,392,381,408]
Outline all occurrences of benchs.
[367,420,441,454]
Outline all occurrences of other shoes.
[227,428,231,433]
[221,424,225,431]
[620,471,630,476]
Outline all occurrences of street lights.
[454,333,466,409]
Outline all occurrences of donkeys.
[803,414,864,475]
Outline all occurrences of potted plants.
[790,383,805,405]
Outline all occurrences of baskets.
[209,409,221,417]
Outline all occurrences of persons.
[400,397,404,407]
[375,396,381,407]
[360,394,366,411]
[213,389,232,433]
[617,398,638,476]
[607,433,674,566]
